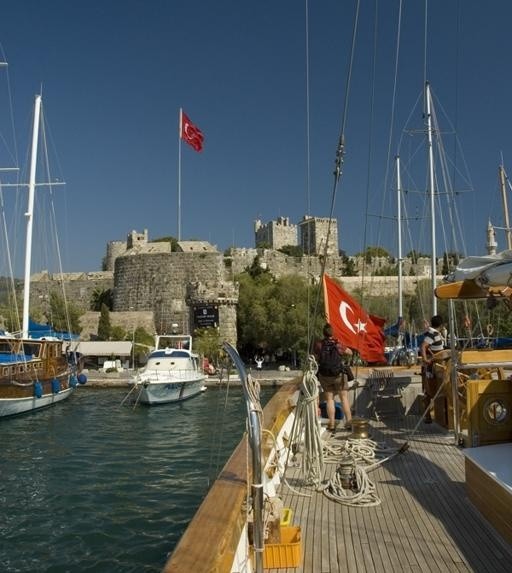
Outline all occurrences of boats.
[128,335,209,404]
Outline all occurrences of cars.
[215,363,227,374]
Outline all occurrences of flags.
[179,112,206,153]
[323,273,388,363]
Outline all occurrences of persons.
[420,316,445,424]
[313,324,353,430]
[255,355,264,379]
[203,355,216,374]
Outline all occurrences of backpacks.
[319,338,342,376]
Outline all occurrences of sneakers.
[328,424,336,430]
[424,415,432,423]
[345,423,351,429]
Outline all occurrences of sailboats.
[0,58,88,416]
[354,80,494,367]
